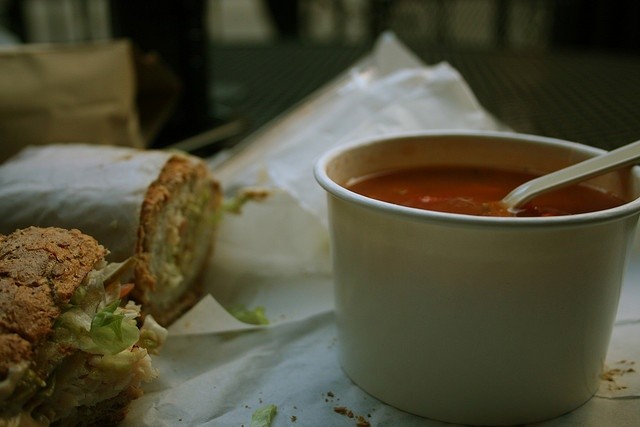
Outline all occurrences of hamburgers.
[1,222,169,427]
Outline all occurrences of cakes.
[2,145,221,328]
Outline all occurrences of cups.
[313,127,639,425]
